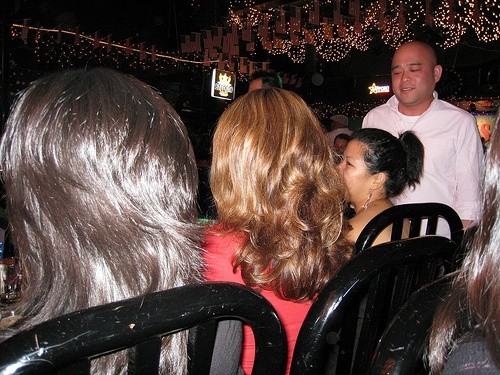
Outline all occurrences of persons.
[360,40,484,238]
[430,110,500,375]
[0,70,209,375]
[335,127,425,259]
[325,114,354,150]
[333,134,351,155]
[197,87,357,375]
[248,70,282,93]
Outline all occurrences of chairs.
[0,204,480,375]
